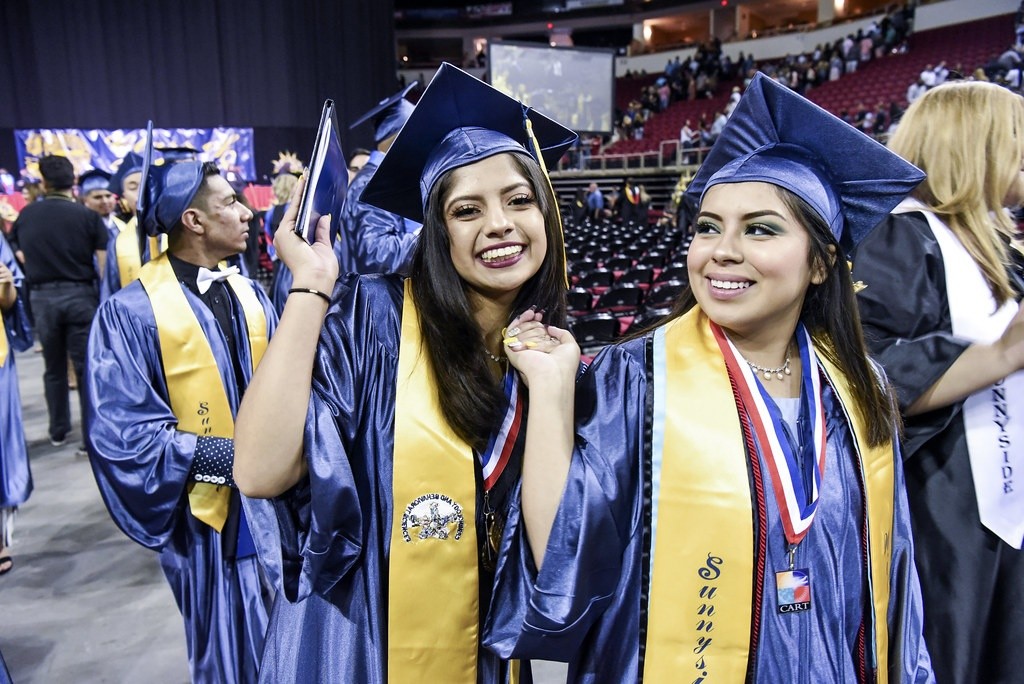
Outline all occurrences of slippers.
[0,556,13,573]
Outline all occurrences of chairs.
[558,207,693,351]
[600,25,1014,161]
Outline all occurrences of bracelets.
[288,288,332,303]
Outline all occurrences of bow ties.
[197,264,241,294]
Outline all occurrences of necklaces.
[484,347,508,363]
[747,341,791,380]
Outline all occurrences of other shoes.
[50,426,72,446]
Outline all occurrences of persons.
[232,63,579,684]
[398,74,406,88]
[340,80,424,275]
[264,157,303,317]
[9,154,109,455]
[79,151,168,304]
[234,190,259,279]
[482,72,936,684]
[0,228,35,573]
[419,73,424,83]
[576,174,650,223]
[566,0,915,170]
[851,80,1024,684]
[477,50,485,67]
[841,22,1024,135]
[84,119,279,684]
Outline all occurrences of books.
[294,98,349,249]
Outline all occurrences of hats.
[138,120,205,257]
[348,80,418,143]
[77,167,116,197]
[358,61,578,291]
[680,72,925,261]
[108,151,144,197]
